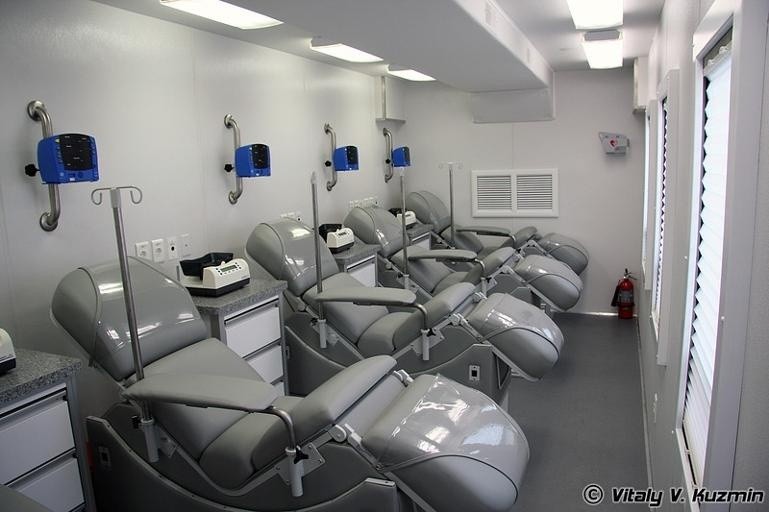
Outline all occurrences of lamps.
[389,64,435,83]
[158,0,285,33]
[564,0,627,32]
[306,35,383,65]
[577,27,625,71]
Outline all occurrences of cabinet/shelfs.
[186,275,291,398]
[328,241,381,292]
[402,219,435,257]
[0,346,100,512]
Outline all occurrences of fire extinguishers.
[617,268,638,319]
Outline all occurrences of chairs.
[47,253,534,512]
[236,216,567,410]
[340,202,585,320]
[404,190,591,276]
